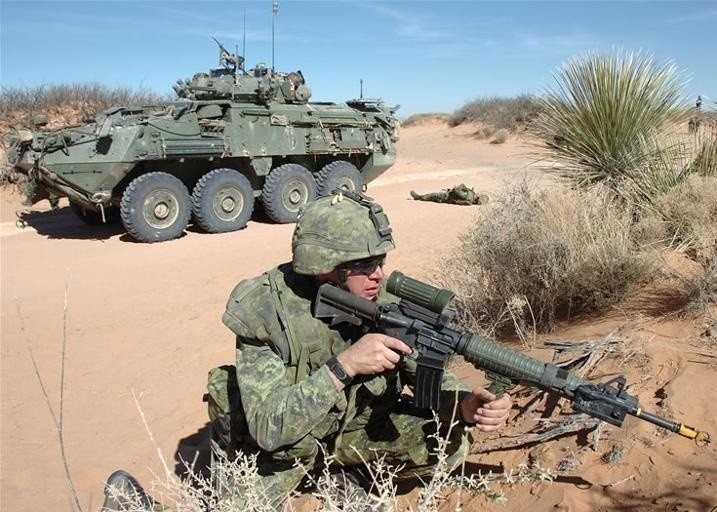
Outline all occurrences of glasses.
[348,256,386,275]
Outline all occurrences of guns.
[314,271,713,448]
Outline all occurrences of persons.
[202,185,515,512]
[19,114,60,215]
[409,185,489,206]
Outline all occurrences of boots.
[22,195,34,206]
[101,470,169,512]
[51,205,58,210]
[411,190,421,200]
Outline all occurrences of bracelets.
[326,355,354,385]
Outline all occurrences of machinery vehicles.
[3,35,402,244]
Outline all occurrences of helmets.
[34,115,48,125]
[291,192,395,276]
[478,192,488,204]
